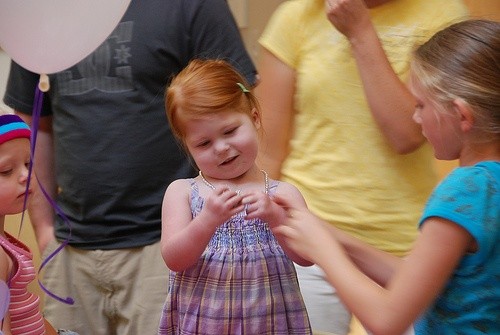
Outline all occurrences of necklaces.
[199,170,268,204]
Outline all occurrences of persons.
[158,55,317,335]
[273,16,500,335]
[2,0,261,334]
[0,104,59,335]
[257,0,471,335]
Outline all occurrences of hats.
[0,114,33,145]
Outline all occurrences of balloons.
[1,0,131,93]
